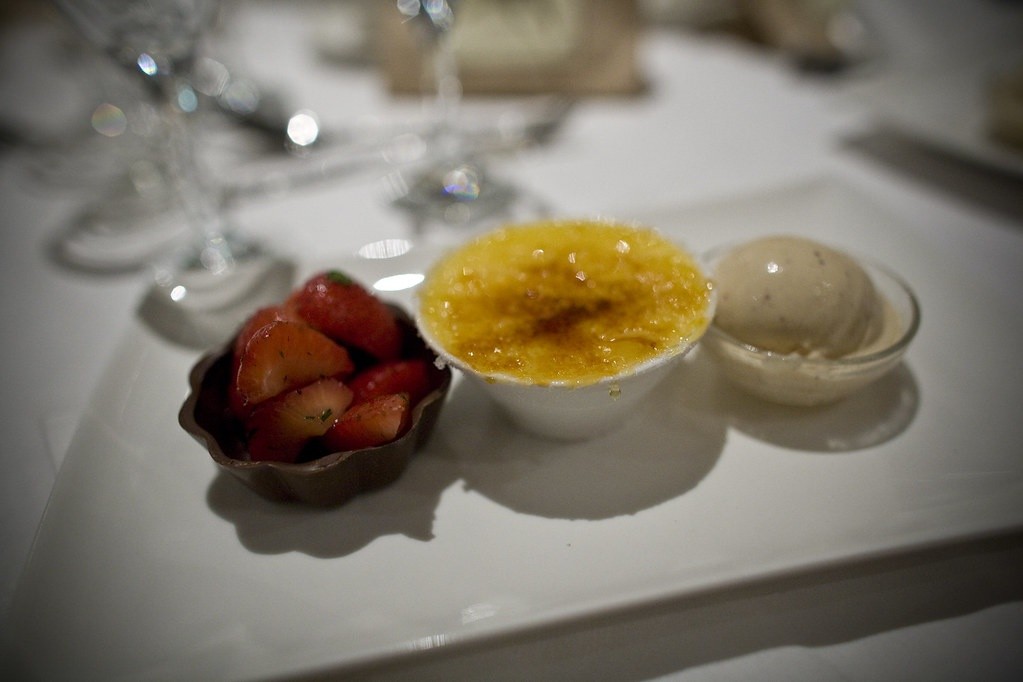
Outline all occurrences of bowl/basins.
[421,219,718,443]
[173,332,450,513]
[691,239,923,410]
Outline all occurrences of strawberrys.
[223,271,428,462]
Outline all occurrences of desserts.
[701,233,902,362]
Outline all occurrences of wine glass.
[26,0,518,351]
